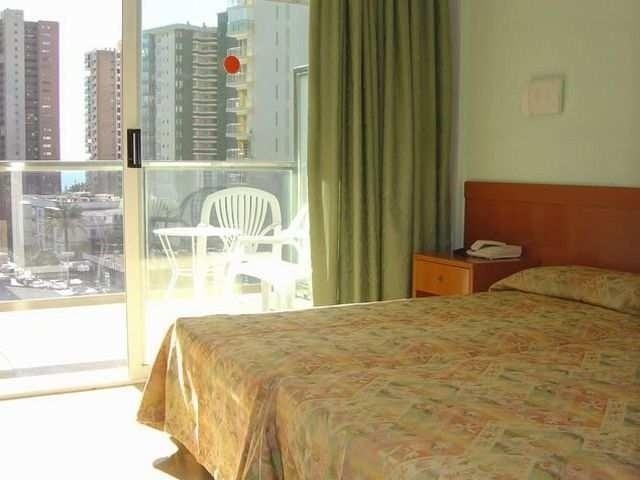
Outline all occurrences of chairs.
[196,187,284,312]
[222,201,313,312]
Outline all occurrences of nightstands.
[411,251,543,299]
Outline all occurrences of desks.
[151,226,240,298]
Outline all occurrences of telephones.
[465,239,523,259]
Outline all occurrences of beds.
[135,292,640,480]
[273,331,640,480]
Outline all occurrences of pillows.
[488,265,639,316]
